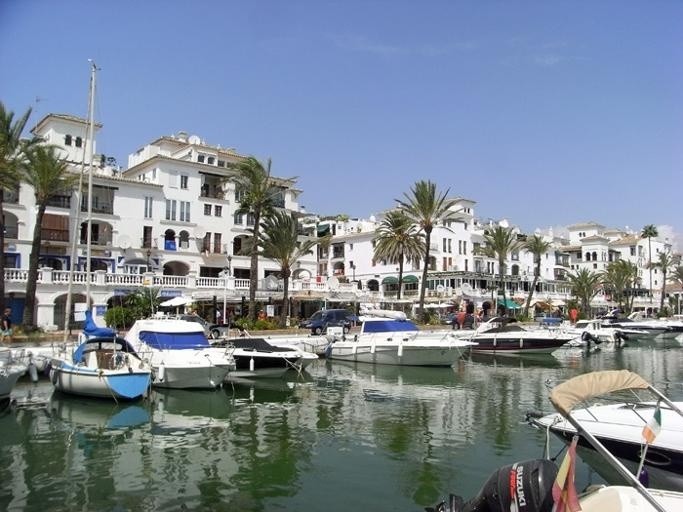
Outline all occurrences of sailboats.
[48,59,155,403]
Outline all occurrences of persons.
[295,302,346,320]
[0,306,14,341]
[215,307,223,325]
[227,309,234,326]
[451,313,468,330]
[459,302,466,313]
[569,306,577,322]
[610,305,623,318]
[595,305,607,320]
[257,309,267,320]
[475,305,485,321]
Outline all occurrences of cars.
[175,315,223,340]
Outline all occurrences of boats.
[456,315,575,356]
[208,323,319,376]
[424,368,682,512]
[556,309,683,348]
[121,285,236,391]
[325,299,479,367]
[0,346,52,396]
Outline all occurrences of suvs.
[298,308,352,335]
